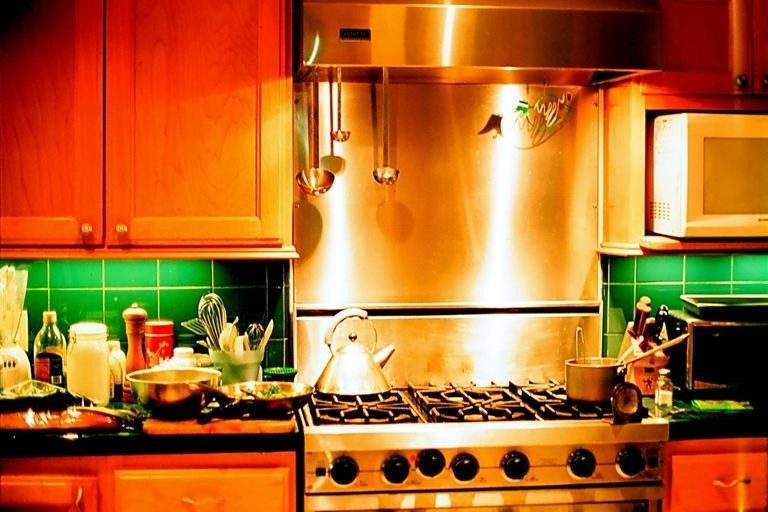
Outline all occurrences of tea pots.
[315,306,398,398]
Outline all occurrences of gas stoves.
[298,379,652,425]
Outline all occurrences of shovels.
[181,318,215,351]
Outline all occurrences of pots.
[565,354,628,402]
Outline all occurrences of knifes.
[631,294,672,356]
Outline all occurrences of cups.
[264,366,297,382]
[209,347,265,386]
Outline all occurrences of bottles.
[65,321,112,407]
[123,302,148,403]
[33,310,67,388]
[654,367,675,407]
[171,346,197,369]
[107,339,127,402]
[143,319,176,368]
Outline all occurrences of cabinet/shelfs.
[600,0,768,256]
[1,0,301,262]
[0,440,301,511]
[663,436,766,512]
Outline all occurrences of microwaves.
[667,306,768,399]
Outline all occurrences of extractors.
[296,2,662,88]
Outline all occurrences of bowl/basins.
[128,368,224,422]
[216,380,313,420]
